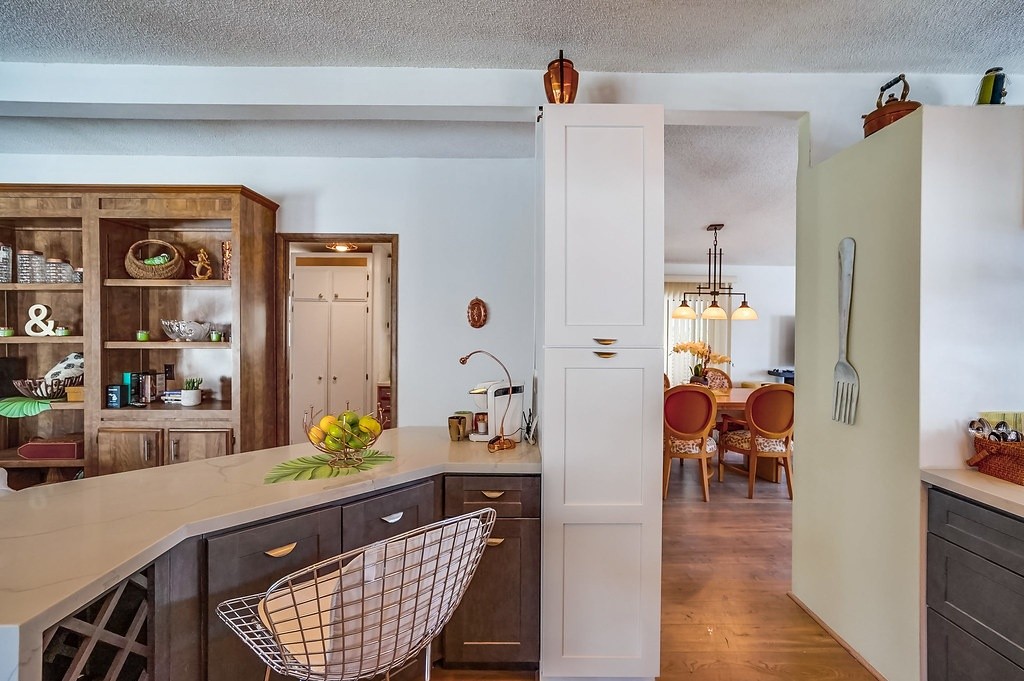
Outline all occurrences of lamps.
[459,350,515,453]
[326,242,359,252]
[673,224,757,320]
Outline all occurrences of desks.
[709,388,778,482]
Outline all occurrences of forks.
[831,238,860,425]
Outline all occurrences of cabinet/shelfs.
[443,474,539,664]
[205,506,342,681]
[289,252,375,445]
[0,183,88,494]
[928,486,1023,681]
[88,188,280,477]
[342,478,435,681]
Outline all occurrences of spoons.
[969,419,1024,442]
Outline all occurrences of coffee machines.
[469,379,524,443]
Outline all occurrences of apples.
[324,411,372,451]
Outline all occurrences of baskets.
[966,436,1024,486]
[125,238,185,279]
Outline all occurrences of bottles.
[0,242,12,284]
[17,250,83,283]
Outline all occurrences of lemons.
[308,411,381,443]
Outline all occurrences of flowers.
[671,340,733,377]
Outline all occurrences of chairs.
[664,385,717,502]
[717,384,794,499]
[216,506,496,681]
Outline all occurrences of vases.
[690,376,708,385]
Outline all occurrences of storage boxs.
[968,438,1024,486]
[123,371,166,403]
[106,384,129,408]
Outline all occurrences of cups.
[55,326,70,337]
[474,412,488,429]
[454,411,473,437]
[137,331,150,341]
[448,416,466,441]
[0,326,14,336]
[210,331,222,342]
[477,420,488,434]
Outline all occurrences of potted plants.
[180,378,203,406]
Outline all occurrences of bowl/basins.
[161,319,211,342]
